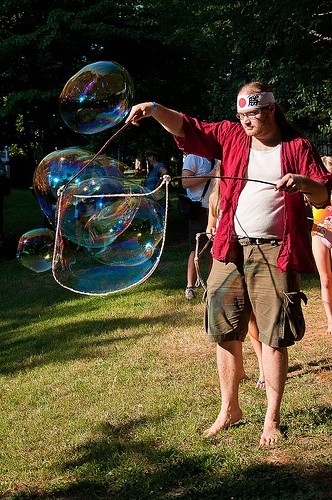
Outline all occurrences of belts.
[236,236,282,245]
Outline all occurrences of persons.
[306,154,332,334]
[182,153,265,390]
[124,82,332,448]
[144,146,171,248]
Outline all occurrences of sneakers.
[184,288,195,300]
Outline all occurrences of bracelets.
[151,102,158,112]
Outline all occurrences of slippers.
[255,379,265,389]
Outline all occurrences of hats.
[144,148,163,156]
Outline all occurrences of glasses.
[236,106,268,120]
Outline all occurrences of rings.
[293,184,297,189]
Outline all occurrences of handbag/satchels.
[180,196,202,220]
